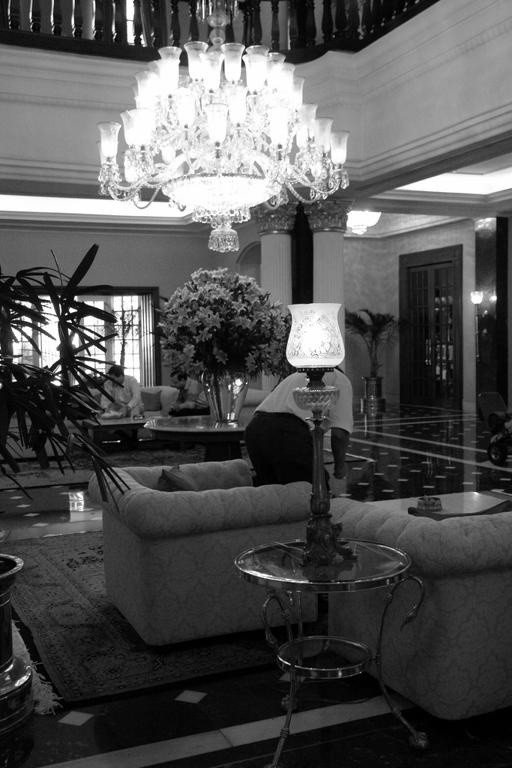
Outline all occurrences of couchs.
[65,385,179,444]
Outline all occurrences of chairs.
[89,456,312,646]
[321,495,512,720]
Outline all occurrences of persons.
[170,370,210,451]
[245,366,353,493]
[100,365,144,450]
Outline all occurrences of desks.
[232,537,427,768]
[142,415,253,464]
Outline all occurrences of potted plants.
[0,243,132,768]
[343,308,400,399]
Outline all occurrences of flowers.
[148,267,301,389]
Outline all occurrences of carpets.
[0,424,367,489]
[0,528,338,705]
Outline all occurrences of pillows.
[140,390,162,412]
[154,468,195,491]
[408,500,512,520]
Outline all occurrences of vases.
[199,369,250,423]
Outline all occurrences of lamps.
[471,290,484,317]
[284,302,346,566]
[97,0,349,253]
[346,209,382,234]
